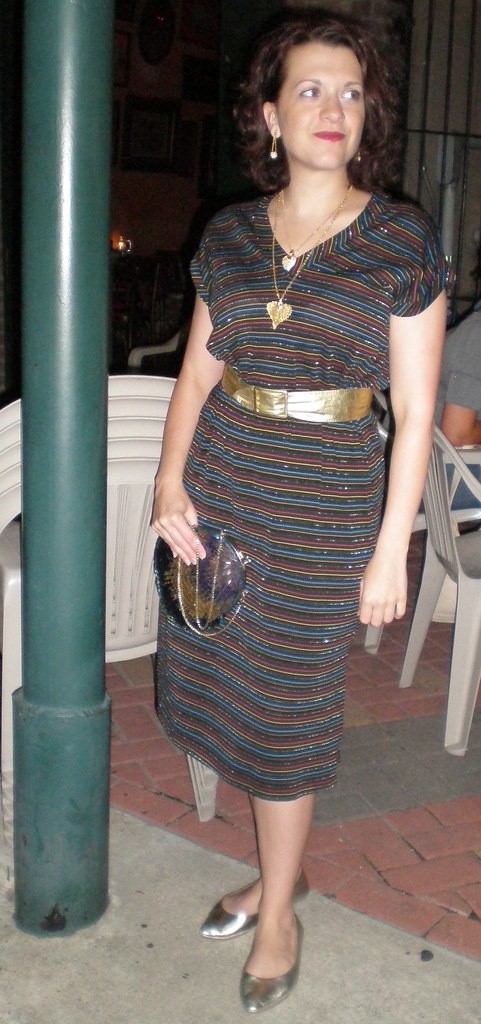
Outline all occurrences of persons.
[439,302,481,522]
[150,13,455,1012]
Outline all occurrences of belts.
[222,364,372,423]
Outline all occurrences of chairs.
[370,388,481,756]
[0,376,218,837]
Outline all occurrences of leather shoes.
[239,913,305,1014]
[198,867,307,940]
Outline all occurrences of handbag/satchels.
[153,522,251,637]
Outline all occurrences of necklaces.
[266,183,354,330]
